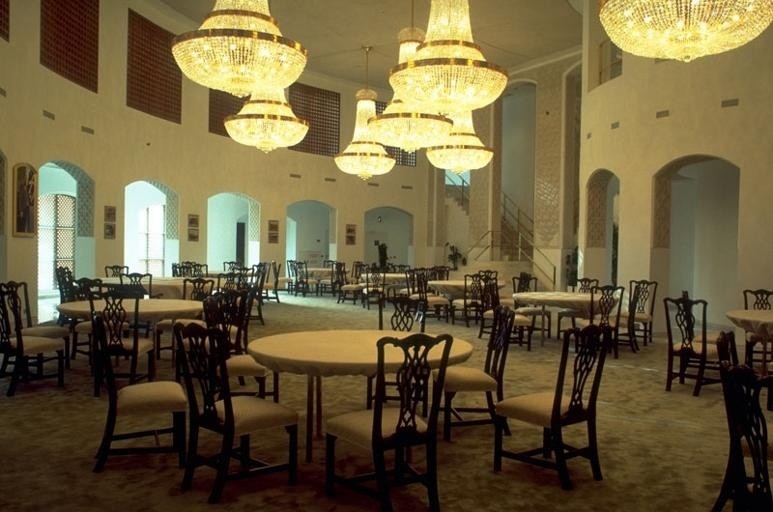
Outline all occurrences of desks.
[246,328,473,466]
[427,279,505,322]
[57,298,203,395]
[82,277,231,298]
[512,292,618,347]
[724,309,773,373]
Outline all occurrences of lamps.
[597,0,773,64]
[366,0,454,153]
[334,49,396,181]
[170,2,308,99]
[387,0,509,117]
[425,109,494,173]
[224,91,309,154]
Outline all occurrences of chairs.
[400,266,450,323]
[557,278,599,339]
[0,290,65,396]
[709,330,773,512]
[479,270,497,277]
[480,277,532,351]
[214,291,241,295]
[170,261,256,276]
[492,325,614,489]
[242,260,281,295]
[118,273,163,338]
[173,322,299,504]
[182,289,259,386]
[724,364,773,511]
[214,295,240,322]
[70,278,102,366]
[681,290,739,368]
[335,260,412,309]
[662,297,722,396]
[500,272,532,305]
[202,295,279,403]
[377,296,427,333]
[83,282,153,396]
[322,334,453,512]
[431,305,515,441]
[249,295,259,321]
[104,265,128,278]
[216,273,241,291]
[511,276,551,339]
[92,316,188,473]
[286,260,346,297]
[576,285,625,359]
[743,289,773,366]
[615,279,658,347]
[609,285,641,354]
[155,278,214,362]
[259,295,279,322]
[0,281,71,375]
[260,322,264,325]
[477,275,498,339]
[55,266,72,326]
[452,274,482,327]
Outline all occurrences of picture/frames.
[12,162,37,238]
